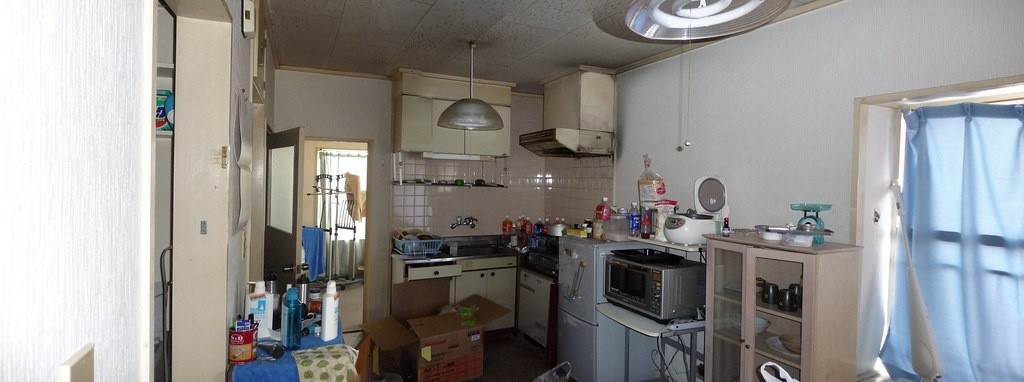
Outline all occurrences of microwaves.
[603,254,706,325]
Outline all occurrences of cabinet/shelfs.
[455,256,516,330]
[391,257,462,317]
[391,68,511,156]
[702,232,863,382]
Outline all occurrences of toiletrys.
[321,280,339,341]
[281,287,302,351]
[244,280,274,339]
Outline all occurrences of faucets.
[449,216,479,229]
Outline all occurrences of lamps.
[626,0,791,147]
[436,41,504,131]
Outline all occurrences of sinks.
[440,244,519,257]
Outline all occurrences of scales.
[790,203,831,244]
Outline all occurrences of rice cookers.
[663,177,730,247]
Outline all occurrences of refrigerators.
[557,234,661,382]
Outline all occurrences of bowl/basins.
[729,314,770,337]
[398,229,435,249]
[778,334,801,354]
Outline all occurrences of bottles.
[307,290,322,313]
[593,195,677,242]
[266,280,280,330]
[502,215,565,249]
[322,280,339,342]
[280,287,302,351]
[297,273,309,303]
[722,218,731,236]
[573,219,592,239]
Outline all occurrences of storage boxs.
[358,275,511,382]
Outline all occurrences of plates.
[766,336,800,358]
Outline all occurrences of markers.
[403,180,416,183]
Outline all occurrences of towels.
[361,191,366,217]
[344,171,363,223]
[290,343,360,382]
[301,227,326,282]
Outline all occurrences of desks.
[596,301,705,382]
[226,317,359,382]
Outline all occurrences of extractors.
[519,128,614,158]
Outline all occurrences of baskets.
[393,236,443,255]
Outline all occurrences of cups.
[789,284,802,308]
[778,289,797,311]
[762,283,779,304]
[455,179,463,185]
[438,180,447,184]
[475,179,485,185]
[228,323,259,365]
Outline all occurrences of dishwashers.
[517,269,554,347]
